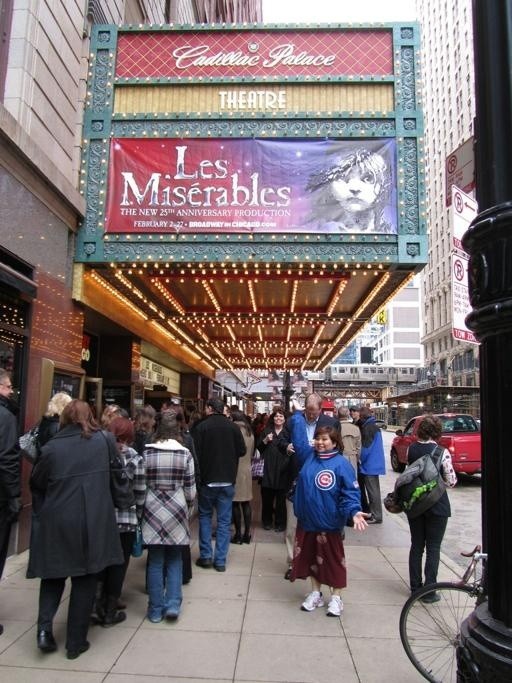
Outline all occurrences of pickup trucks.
[390,413,481,479]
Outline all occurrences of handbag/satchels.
[108,458,136,511]
[17,425,40,464]
[250,447,265,481]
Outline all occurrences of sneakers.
[300,591,325,612]
[212,562,227,573]
[326,593,344,617]
[195,557,213,568]
[284,568,291,579]
[263,523,286,531]
[421,592,441,604]
[88,603,128,629]
[165,609,180,618]
[151,616,163,622]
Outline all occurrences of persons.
[406,415,458,603]
[1,369,387,659]
[305,141,392,235]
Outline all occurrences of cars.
[373,419,387,429]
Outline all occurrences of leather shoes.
[365,518,382,524]
[64,639,92,659]
[36,628,58,653]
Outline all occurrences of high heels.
[243,531,252,545]
[229,534,243,545]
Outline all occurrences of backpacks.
[389,442,447,521]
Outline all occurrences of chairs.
[452,420,466,430]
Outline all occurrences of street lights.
[425,369,437,386]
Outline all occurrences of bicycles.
[398,544,490,682]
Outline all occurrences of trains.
[257,362,420,385]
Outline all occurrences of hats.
[271,404,286,415]
